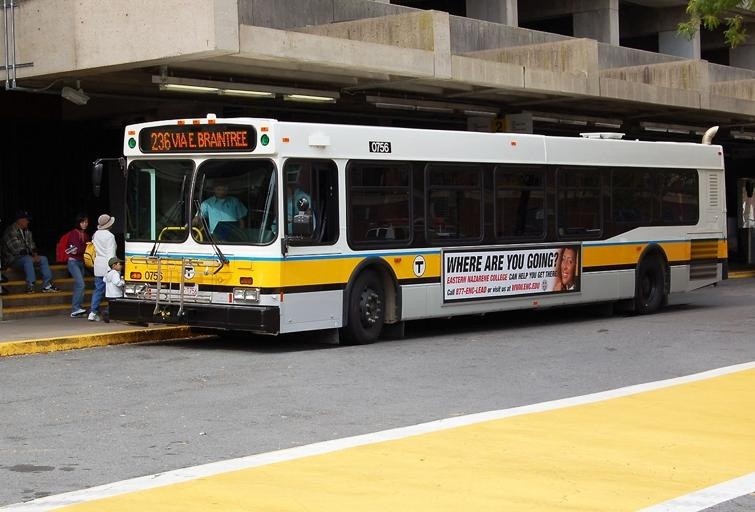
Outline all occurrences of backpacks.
[83,239,96,268]
[54,230,71,263]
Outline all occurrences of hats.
[96,213,116,230]
[108,256,125,267]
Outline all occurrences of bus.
[108,114,729,347]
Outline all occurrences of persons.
[3,209,62,294]
[101,256,125,323]
[87,214,117,322]
[185,180,247,242]
[67,215,90,318]
[271,183,316,236]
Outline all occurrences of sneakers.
[42,280,64,293]
[23,282,36,294]
[88,311,100,322]
[70,307,87,318]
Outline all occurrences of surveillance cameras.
[61,86,91,105]
